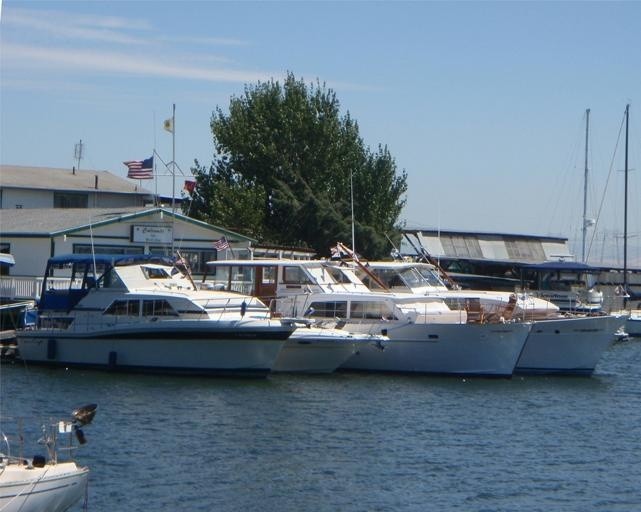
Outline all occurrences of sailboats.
[566,103,641,339]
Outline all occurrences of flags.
[124,155,154,180]
[163,117,174,133]
[176,258,193,279]
[213,235,230,253]
[339,243,359,264]
[182,180,196,195]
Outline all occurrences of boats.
[272,316,395,376]
[198,172,536,378]
[0,401,102,512]
[10,104,313,378]
[382,229,631,378]
